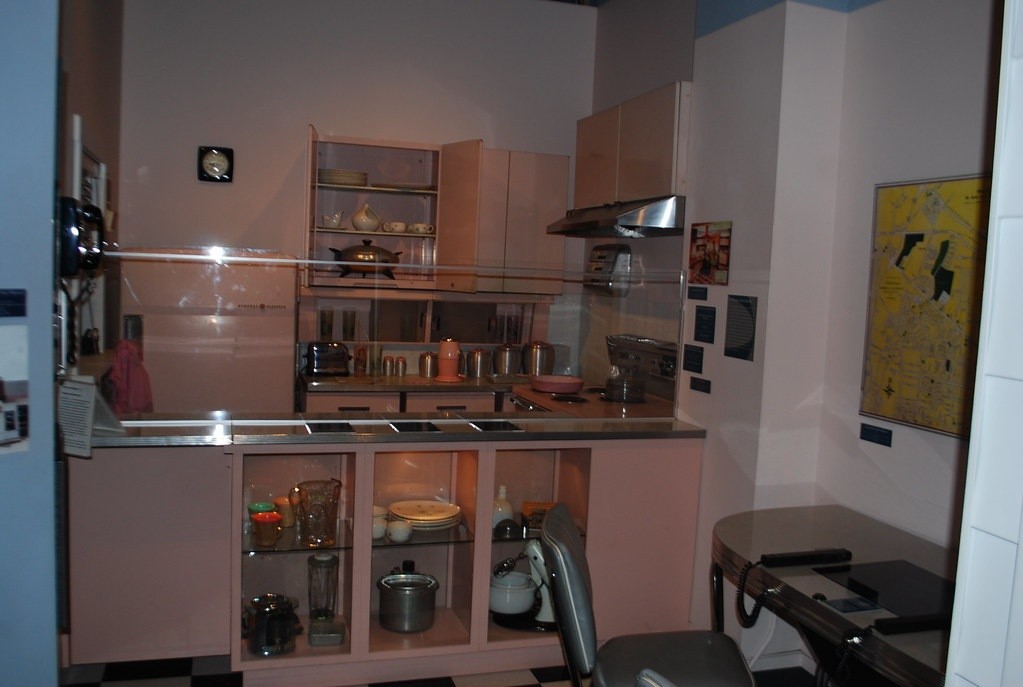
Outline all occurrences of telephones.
[59,197,102,275]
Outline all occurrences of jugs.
[289,478,342,547]
[352,203,380,231]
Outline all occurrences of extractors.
[546,195,685,238]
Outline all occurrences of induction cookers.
[512,383,674,418]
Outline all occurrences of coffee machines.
[490,539,556,633]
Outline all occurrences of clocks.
[196,145,236,184]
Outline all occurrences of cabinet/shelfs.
[301,122,570,348]
[296,379,517,413]
[574,81,692,210]
[65,439,716,687]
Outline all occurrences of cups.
[384,222,406,232]
[322,210,343,228]
[387,521,415,543]
[275,497,297,529]
[412,224,434,233]
[372,519,386,539]
[247,501,276,533]
[251,513,280,545]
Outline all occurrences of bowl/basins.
[530,376,584,392]
[494,519,521,539]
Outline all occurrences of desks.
[708,504,959,687]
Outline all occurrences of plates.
[374,506,388,519]
[390,500,462,531]
[318,168,368,186]
[371,181,434,191]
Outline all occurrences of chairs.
[539,500,756,685]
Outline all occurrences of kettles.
[604,370,648,400]
[242,593,299,657]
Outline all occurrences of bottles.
[383,357,394,376]
[307,554,337,620]
[522,341,554,376]
[466,348,492,378]
[395,357,407,376]
[419,352,437,378]
[493,344,522,376]
[494,485,513,529]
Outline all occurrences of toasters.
[303,341,353,376]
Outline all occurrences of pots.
[329,239,403,279]
[376,560,440,633]
[493,571,536,613]
